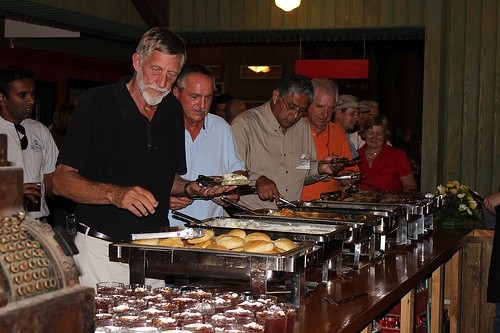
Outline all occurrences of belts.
[75,223,119,243]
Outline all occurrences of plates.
[196,180,252,186]
[310,159,348,164]
[330,175,361,179]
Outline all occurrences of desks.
[168,229,477,333]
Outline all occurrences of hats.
[333,95,370,113]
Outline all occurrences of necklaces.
[365,148,380,160]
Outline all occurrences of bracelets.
[182,180,194,201]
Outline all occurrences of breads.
[132,229,297,253]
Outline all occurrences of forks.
[323,291,368,306]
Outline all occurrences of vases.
[435,207,468,231]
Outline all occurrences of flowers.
[436,180,484,216]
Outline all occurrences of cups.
[24,181,41,213]
[92,281,297,333]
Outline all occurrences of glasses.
[279,95,306,113]
[14,123,27,150]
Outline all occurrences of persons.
[214,94,231,118]
[484,189,500,333]
[334,94,361,159]
[0,67,59,222]
[300,78,361,200]
[229,73,345,205]
[348,100,381,150]
[225,99,248,124]
[50,26,238,295]
[167,63,249,227]
[356,116,417,194]
[48,104,73,142]
